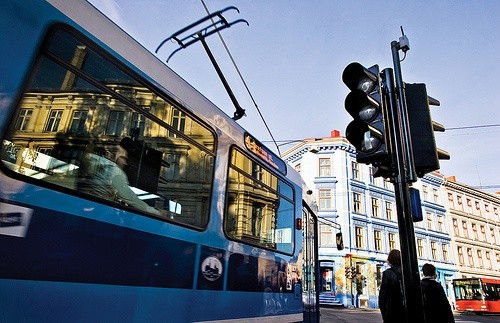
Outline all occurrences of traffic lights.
[343,22,451,321]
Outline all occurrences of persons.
[420,264,456,323]
[75,143,171,220]
[379,250,405,323]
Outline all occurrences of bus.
[451,278,500,316]
[1,0,343,323]
